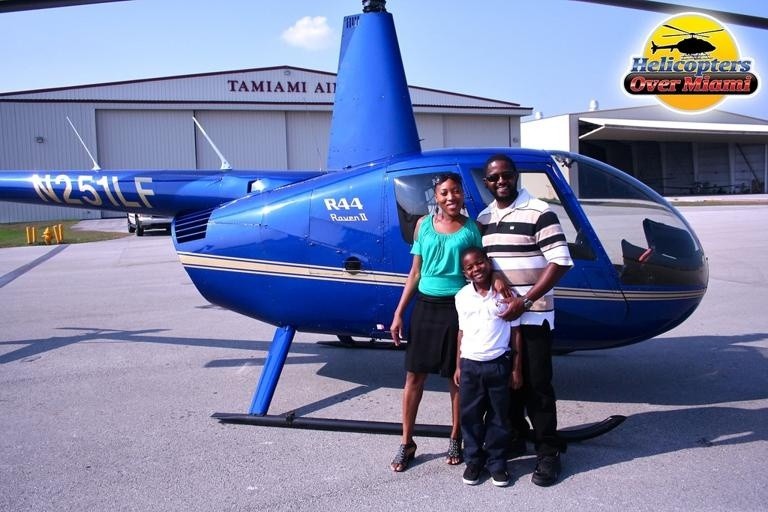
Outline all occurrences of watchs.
[521,295,534,311]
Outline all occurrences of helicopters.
[1,0,768,444]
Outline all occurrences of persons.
[473,154,574,486]
[453,245,522,486]
[385,173,515,473]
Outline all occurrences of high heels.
[447,435,464,467]
[390,441,417,471]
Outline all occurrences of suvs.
[126,212,174,238]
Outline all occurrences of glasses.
[486,171,517,181]
[435,172,464,184]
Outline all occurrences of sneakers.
[491,461,513,487]
[531,449,561,487]
[463,460,481,485]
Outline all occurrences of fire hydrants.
[43,228,52,245]
